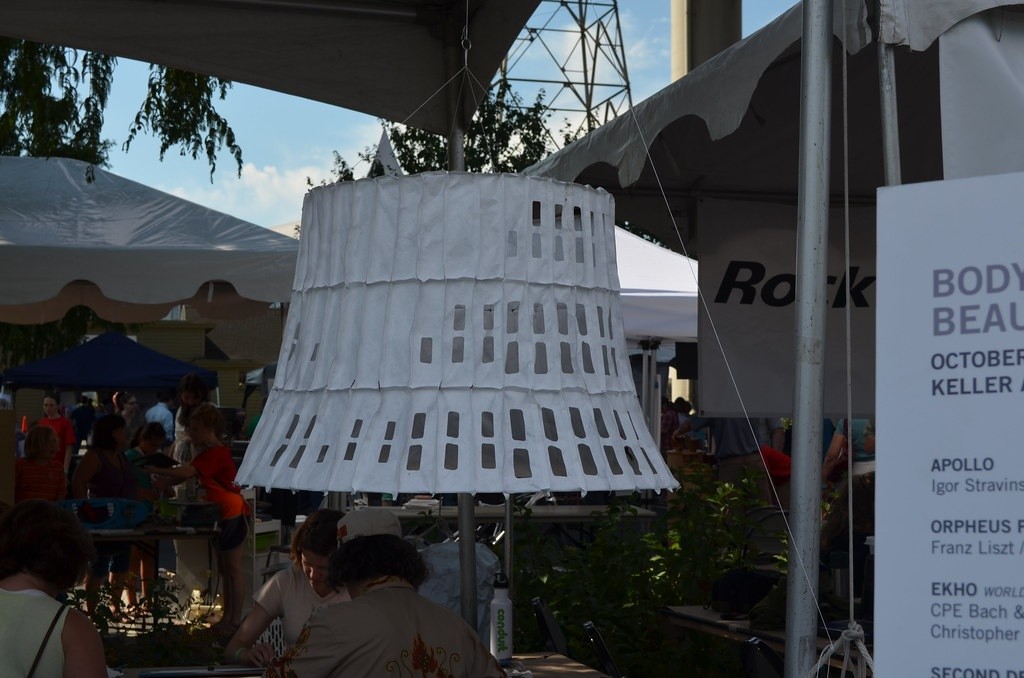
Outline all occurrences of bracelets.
[234,647,247,665]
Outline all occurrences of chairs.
[533,597,785,678]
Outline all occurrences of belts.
[719,450,759,460]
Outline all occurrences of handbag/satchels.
[828,417,855,483]
[703,567,774,620]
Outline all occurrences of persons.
[65,373,255,625]
[14,425,66,508]
[36,392,76,475]
[224,509,352,669]
[654,394,876,615]
[261,507,507,678]
[0,398,8,410]
[0,499,109,678]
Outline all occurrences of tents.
[613,225,699,448]
[0,157,303,343]
[519,0,1024,263]
[0,328,221,410]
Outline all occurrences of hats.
[337,508,402,549]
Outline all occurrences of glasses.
[126,401,138,405]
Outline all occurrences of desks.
[123,652,610,678]
[87,527,219,607]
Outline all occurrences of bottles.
[490,573,512,664]
[382,493,392,506]
